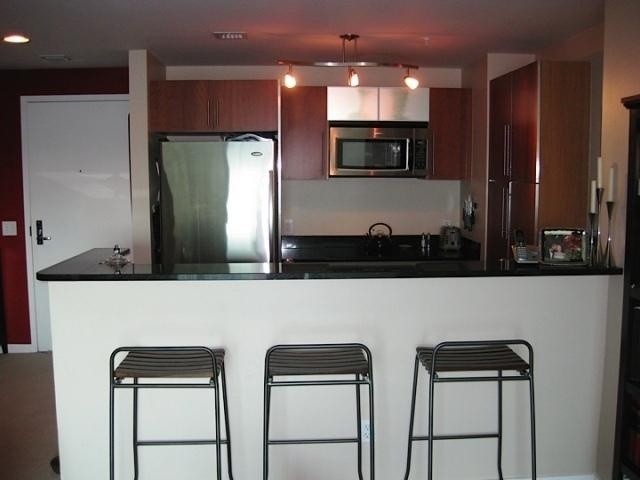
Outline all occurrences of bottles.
[421,233,431,248]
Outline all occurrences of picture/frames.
[538,229,587,266]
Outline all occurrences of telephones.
[512,229,538,264]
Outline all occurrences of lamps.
[277,35,419,90]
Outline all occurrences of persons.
[544,235,577,262]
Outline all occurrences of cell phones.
[513,227,529,262]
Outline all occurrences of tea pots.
[366,223,393,256]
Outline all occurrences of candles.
[590,157,615,214]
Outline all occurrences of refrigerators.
[159,133,277,264]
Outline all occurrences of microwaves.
[329,126,434,178]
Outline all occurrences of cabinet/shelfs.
[612,95,640,480]
[488,61,591,261]
[327,86,430,122]
[149,80,278,132]
[281,86,326,180]
[430,87,473,180]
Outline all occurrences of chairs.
[263,344,374,480]
[405,340,536,480]
[110,347,232,480]
